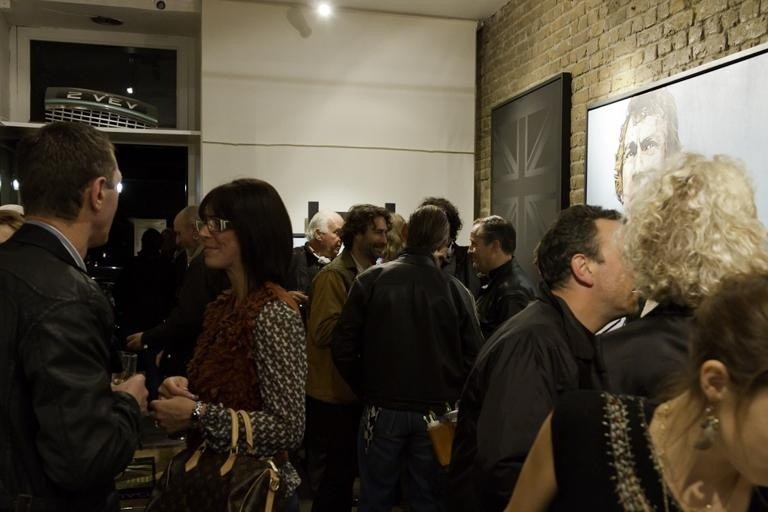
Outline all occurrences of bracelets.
[190,400,202,430]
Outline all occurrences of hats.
[0,204,26,230]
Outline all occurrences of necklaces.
[645,414,748,511]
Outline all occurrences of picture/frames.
[490,68,574,295]
[581,42,766,226]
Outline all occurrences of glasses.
[194,216,232,234]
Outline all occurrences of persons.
[127,207,229,390]
[0,208,26,246]
[284,198,538,510]
[588,150,767,404]
[150,177,309,512]
[606,89,683,206]
[0,116,150,511]
[444,205,650,511]
[501,272,767,511]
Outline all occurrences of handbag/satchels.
[144,406,288,512]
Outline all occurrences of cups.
[110,350,138,386]
